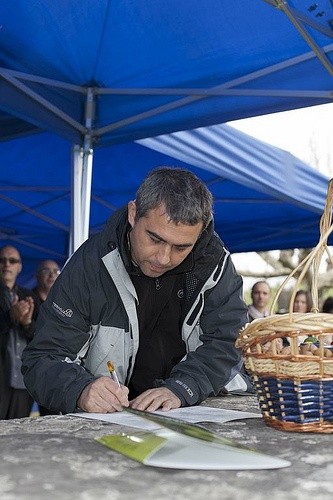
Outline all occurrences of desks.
[1,392,333,500]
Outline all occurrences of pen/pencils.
[107,361,121,388]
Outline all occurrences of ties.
[9,292,14,303]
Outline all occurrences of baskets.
[234,179,333,433]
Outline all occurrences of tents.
[0,0,333,257]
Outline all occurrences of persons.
[248,281,271,323]
[21,167,248,413]
[30,260,61,318]
[0,246,34,420]
[293,290,311,313]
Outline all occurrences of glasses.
[0,257,20,263]
[39,269,61,275]
